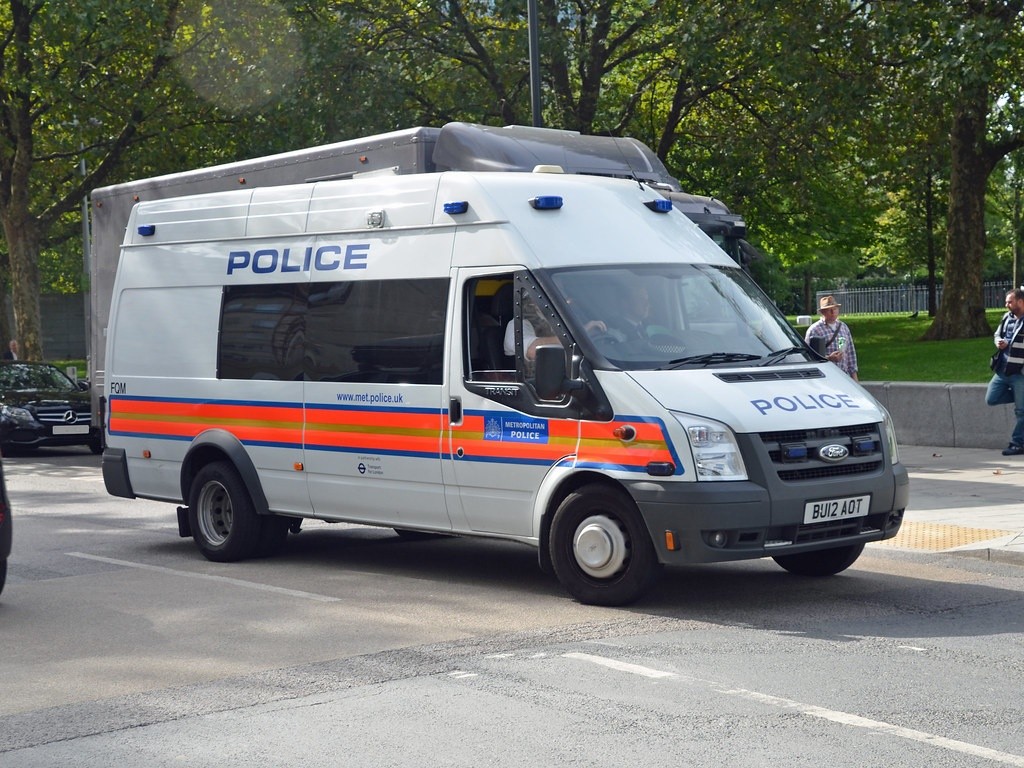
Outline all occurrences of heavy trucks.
[77,119,745,434]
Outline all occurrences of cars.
[0,461,14,594]
[0,358,104,458]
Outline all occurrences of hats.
[817,296,841,312]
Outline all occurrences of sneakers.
[1002,442,1024,455]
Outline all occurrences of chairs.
[482,283,516,370]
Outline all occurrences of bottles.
[838,335,846,352]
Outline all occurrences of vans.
[101,163,910,612]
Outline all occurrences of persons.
[805,296,858,382]
[504,281,608,380]
[607,282,649,344]
[985,289,1024,455]
